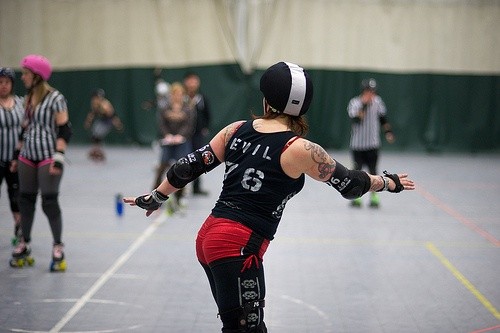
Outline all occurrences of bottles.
[115,193,124,217]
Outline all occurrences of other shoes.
[174,195,184,207]
[191,190,208,197]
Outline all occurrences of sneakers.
[370,193,379,208]
[9,243,34,267]
[50,246,67,272]
[352,197,361,207]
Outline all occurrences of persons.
[0,66,28,247]
[9,54,73,272]
[124,61,414,333]
[346,79,393,207]
[85,89,124,160]
[150,74,211,209]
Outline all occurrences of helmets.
[0,66,16,82]
[260,62,313,117]
[361,78,377,93]
[20,54,52,81]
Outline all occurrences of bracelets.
[385,130,392,134]
[375,175,390,192]
